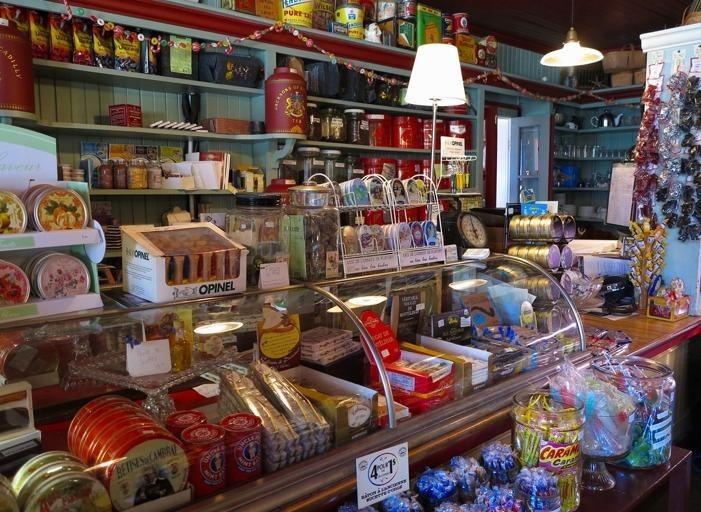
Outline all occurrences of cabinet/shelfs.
[273,19,483,213]
[548,85,643,222]
[1,0,273,290]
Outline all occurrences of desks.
[579,306,701,512]
[582,448,692,510]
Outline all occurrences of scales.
[436,193,488,260]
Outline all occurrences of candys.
[628,218,669,293]
[549,380,635,456]
[593,352,673,471]
[335,444,561,512]
[514,394,580,512]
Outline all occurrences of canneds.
[96,158,162,189]
[166,411,264,501]
[304,103,368,144]
[279,146,365,185]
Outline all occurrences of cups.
[621,116,642,125]
[590,110,600,128]
[554,144,630,159]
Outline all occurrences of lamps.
[539,1,605,68]
[404,43,466,197]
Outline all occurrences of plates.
[554,193,595,218]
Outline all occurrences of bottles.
[279,103,370,185]
[591,355,676,471]
[336,453,562,511]
[509,389,584,512]
[98,158,163,190]
[279,185,340,283]
[225,191,291,286]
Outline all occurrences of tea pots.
[565,121,579,131]
[590,109,624,127]
